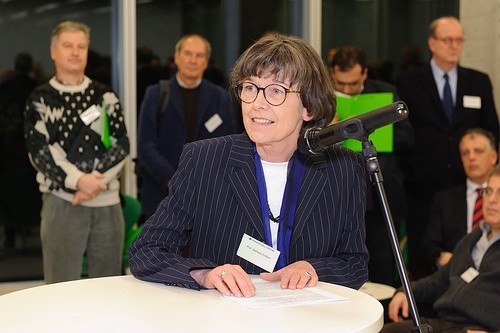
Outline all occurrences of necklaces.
[267,203,280,223]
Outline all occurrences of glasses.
[435,36,464,45]
[235,81,303,107]
[482,187,500,203]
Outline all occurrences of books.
[330,91,393,153]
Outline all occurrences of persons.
[417,128,500,273]
[397,16,500,280]
[132,33,246,228]
[379,163,500,333]
[128,32,370,297]
[324,48,417,288]
[24,21,130,285]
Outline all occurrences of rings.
[305,271,312,277]
[221,270,228,277]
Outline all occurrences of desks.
[0,274,384,333]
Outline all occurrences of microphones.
[296,101,409,155]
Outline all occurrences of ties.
[442,74,454,122]
[471,188,486,232]
